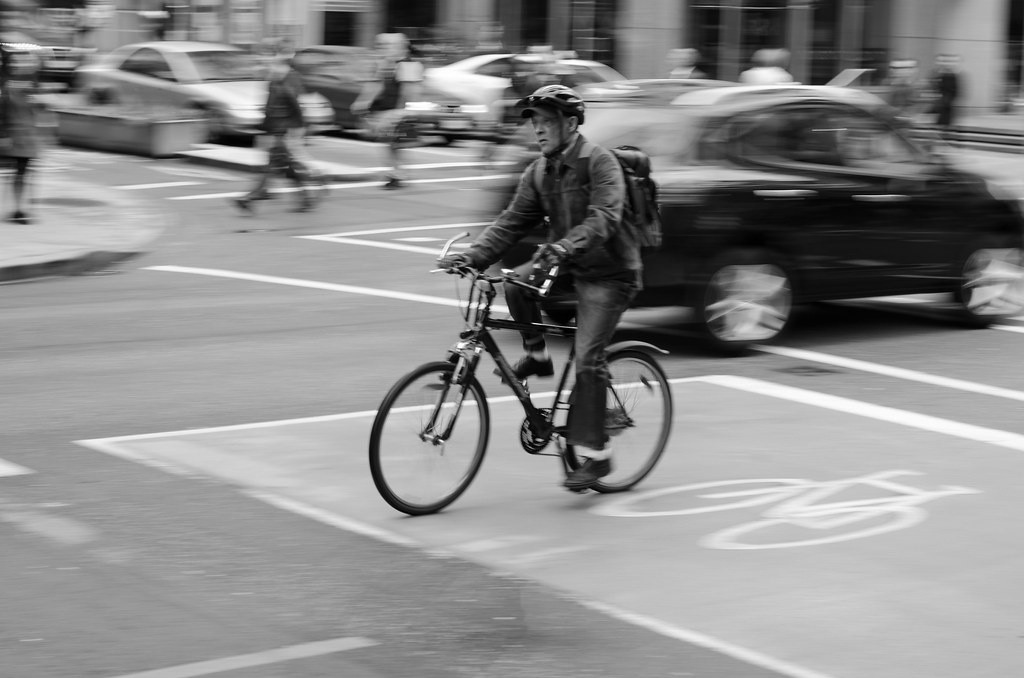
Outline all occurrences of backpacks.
[575,140,663,252]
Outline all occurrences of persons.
[435,84,645,492]
[884,45,968,145]
[1,57,39,222]
[233,59,314,219]
[355,27,426,191]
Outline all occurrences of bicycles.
[367,231,672,516]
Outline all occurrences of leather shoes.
[493,356,555,383]
[563,458,611,489]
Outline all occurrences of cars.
[0,30,93,89]
[422,53,629,143]
[288,46,380,132]
[76,42,281,143]
[495,84,1024,353]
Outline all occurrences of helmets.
[514,84,586,125]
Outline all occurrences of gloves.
[530,243,569,286]
[436,251,477,280]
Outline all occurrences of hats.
[519,106,561,120]
